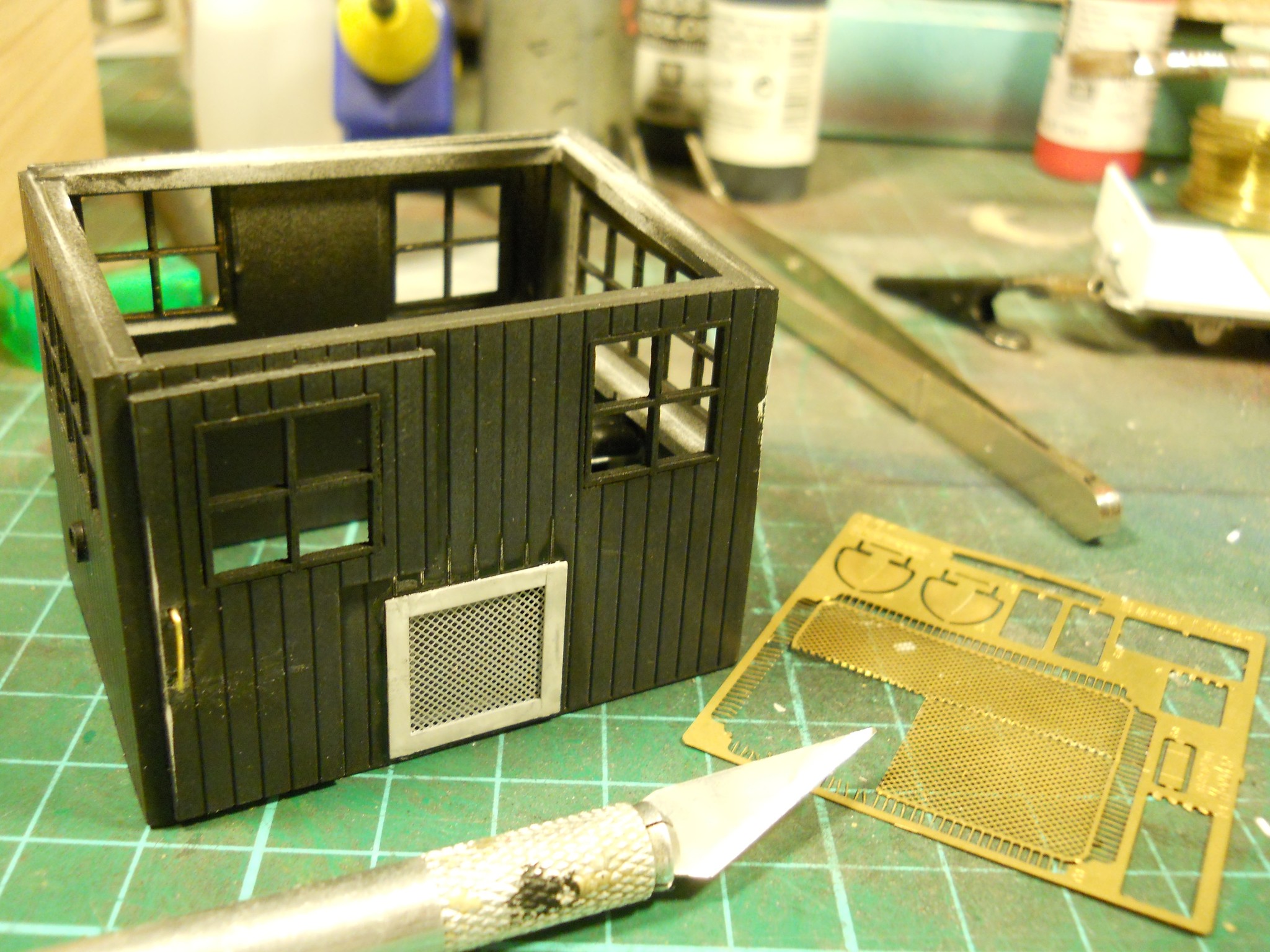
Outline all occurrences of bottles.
[708,0,826,203]
[639,2,708,167]
[1033,1,1174,183]
[475,0,638,216]
